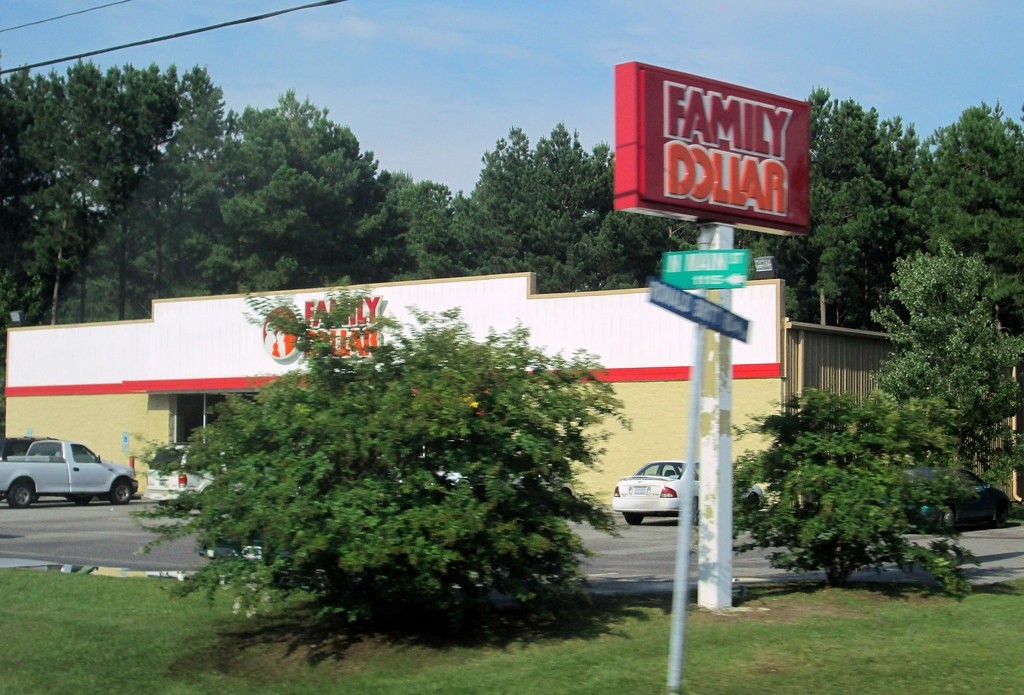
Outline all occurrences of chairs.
[664,470,675,476]
[55,450,61,457]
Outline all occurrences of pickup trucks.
[0,439,140,510]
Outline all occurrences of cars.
[879,467,1015,534]
[0,434,92,462]
[140,443,247,517]
[436,470,576,517]
[612,459,765,525]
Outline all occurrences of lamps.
[9,310,24,327]
[753,256,777,280]
